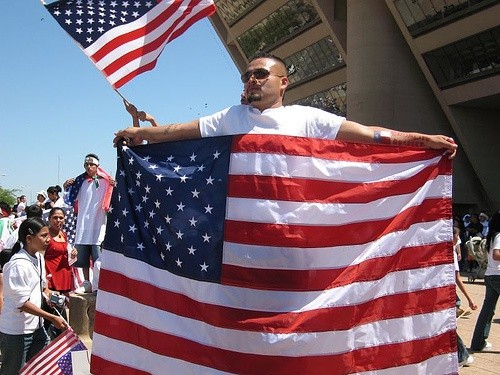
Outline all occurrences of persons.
[0,49,500,375]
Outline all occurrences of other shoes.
[456,307,472,317]
[457,356,474,367]
[470,343,492,350]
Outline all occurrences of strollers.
[465,236,489,284]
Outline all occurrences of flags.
[18,326,89,375]
[44,0,215,90]
[91,134,459,375]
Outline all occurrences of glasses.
[241,69,284,83]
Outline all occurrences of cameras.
[51,293,67,305]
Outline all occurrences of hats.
[37,190,48,199]
[479,212,489,220]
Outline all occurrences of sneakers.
[74,281,93,294]
